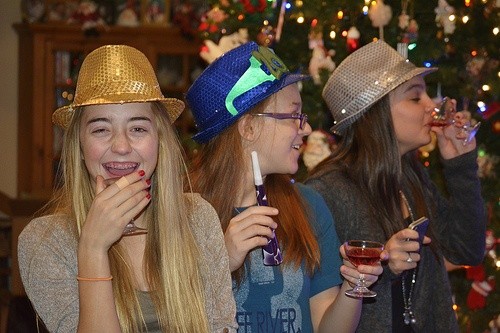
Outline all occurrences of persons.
[17,44,236,333]
[185,41,389,333]
[302,39,487,333]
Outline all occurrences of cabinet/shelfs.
[11,20,202,197]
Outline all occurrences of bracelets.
[77,276,113,281]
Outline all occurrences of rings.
[407,253,412,262]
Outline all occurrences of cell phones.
[406,216,429,263]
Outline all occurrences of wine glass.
[102,176,147,234]
[429,96,481,145]
[345,239,385,298]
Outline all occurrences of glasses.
[249,112,308,130]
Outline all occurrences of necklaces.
[399,190,416,324]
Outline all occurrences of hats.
[322,39,439,136]
[51,45,185,129]
[187,41,312,143]
